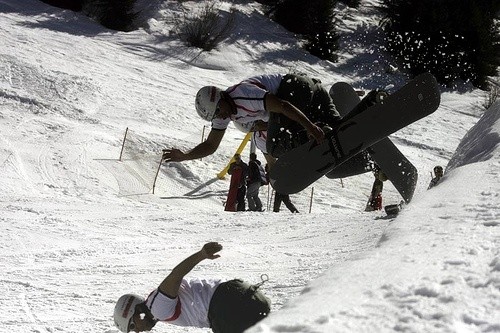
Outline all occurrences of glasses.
[129,317,136,330]
[215,106,220,116]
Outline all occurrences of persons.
[227,153,248,210]
[427,166,443,189]
[246,152,263,211]
[273,188,300,213]
[163,72,343,162]
[113,241,271,332]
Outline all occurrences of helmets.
[195,86,222,121]
[114,293,145,333]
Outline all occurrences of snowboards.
[325,150,378,179]
[268,72,441,194]
[329,81,418,204]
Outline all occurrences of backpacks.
[252,160,269,185]
